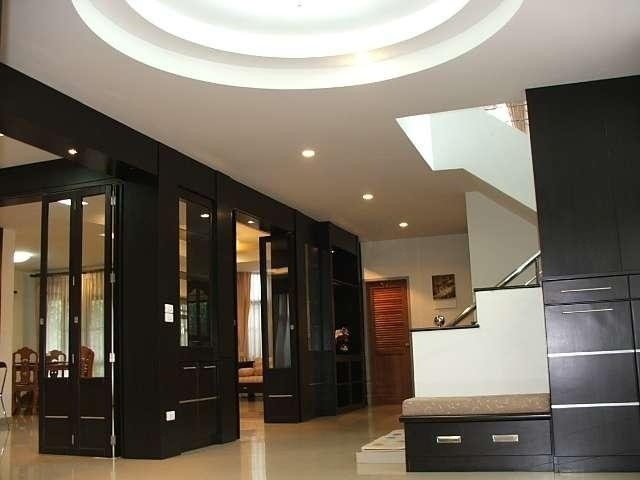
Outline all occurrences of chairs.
[11,346,94,419]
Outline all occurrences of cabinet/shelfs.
[525,75,640,276]
[399,416,553,472]
[541,274,640,473]
[322,222,368,417]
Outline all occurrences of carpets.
[363,422,404,450]
